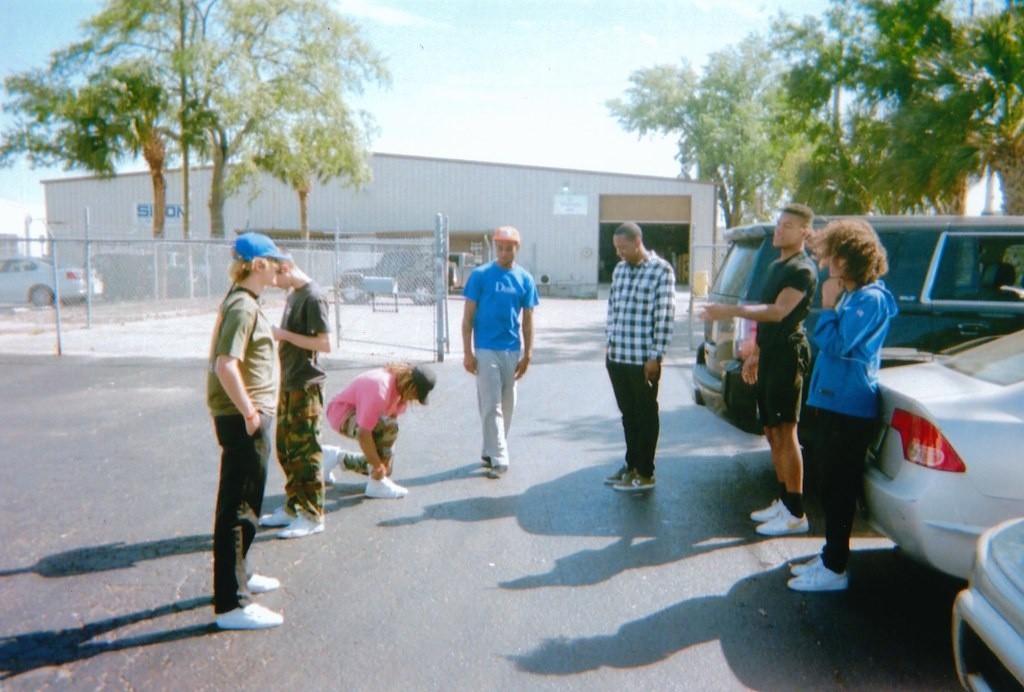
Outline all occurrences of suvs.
[688,213,1023,445]
[340,251,457,307]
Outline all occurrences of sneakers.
[605,464,632,483]
[245,572,280,593]
[789,559,848,592]
[755,511,810,536]
[319,444,341,483]
[276,515,324,540]
[613,468,656,490]
[750,497,786,522]
[365,475,409,498]
[215,602,284,629]
[791,554,823,576]
[261,505,300,526]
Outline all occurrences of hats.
[413,364,436,405]
[493,226,519,243]
[232,233,286,262]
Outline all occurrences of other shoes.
[481,452,508,479]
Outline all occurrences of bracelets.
[244,409,259,421]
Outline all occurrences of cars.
[857,330,1023,577]
[0,256,104,310]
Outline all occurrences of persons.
[260,246,333,539]
[606,222,676,490]
[207,232,291,630]
[461,225,540,479]
[699,208,818,535]
[326,361,436,498]
[787,219,898,590]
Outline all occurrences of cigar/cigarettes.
[648,380,653,387]
